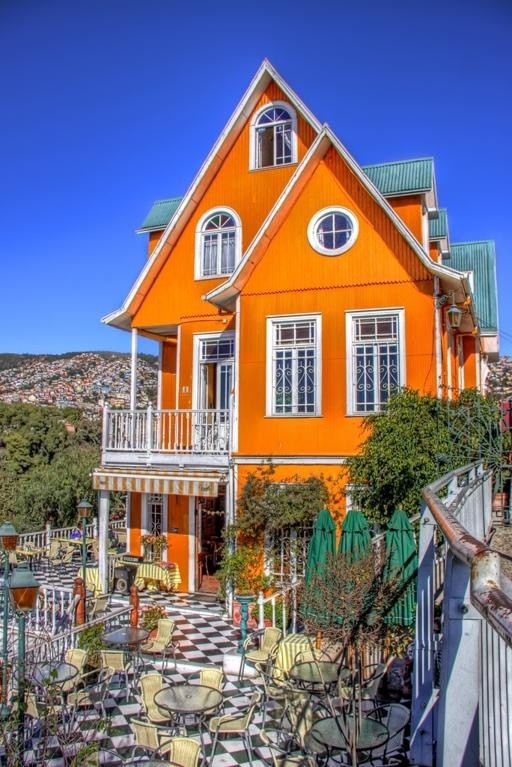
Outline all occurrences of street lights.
[0,521,41,766]
[77,499,94,624]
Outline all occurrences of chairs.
[12,618,412,766]
[10,529,181,627]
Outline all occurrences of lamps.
[437,286,463,332]
